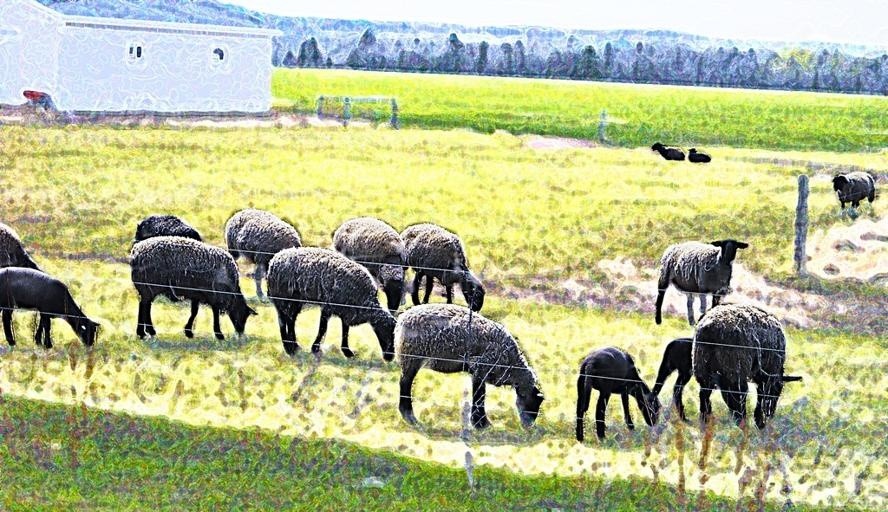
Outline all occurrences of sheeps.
[651,141,686,160]
[0,222,42,271]
[394,303,545,432]
[575,346,662,445]
[688,147,711,163]
[654,238,749,325]
[831,170,876,209]
[127,207,486,361]
[0,265,100,351]
[652,300,803,429]
